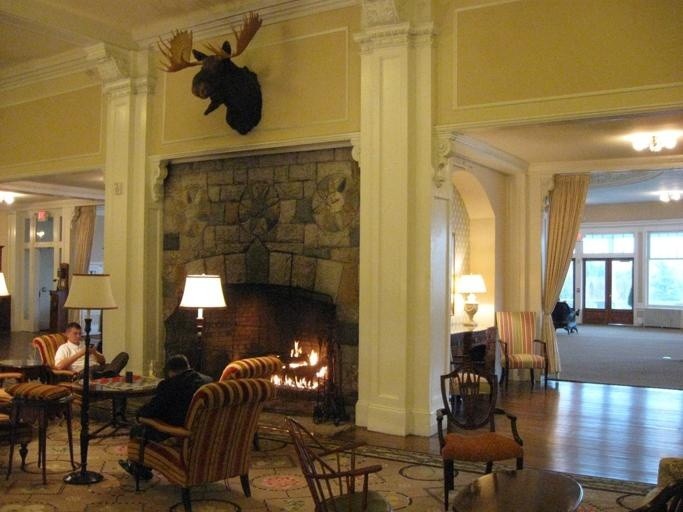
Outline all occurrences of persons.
[117,354,227,482]
[54,322,129,379]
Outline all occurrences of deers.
[152,10,266,135]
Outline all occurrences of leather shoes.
[95,370,116,379]
[112,417,131,426]
[119,459,153,481]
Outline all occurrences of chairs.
[0,370,34,471]
[433,362,524,512]
[551,300,579,335]
[284,414,394,512]
[30,333,120,422]
[624,458,682,512]
[125,377,275,511]
[215,354,283,450]
[494,309,550,391]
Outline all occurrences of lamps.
[455,273,487,328]
[60,270,117,484]
[176,273,228,370]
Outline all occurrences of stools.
[449,372,491,412]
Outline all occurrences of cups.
[125,371,133,384]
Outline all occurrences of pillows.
[5,381,70,402]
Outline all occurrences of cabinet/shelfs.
[48,289,68,331]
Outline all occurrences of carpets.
[0,404,683,512]
[542,319,682,390]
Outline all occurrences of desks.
[450,469,584,512]
[0,357,55,383]
[71,371,165,442]
[2,390,82,487]
